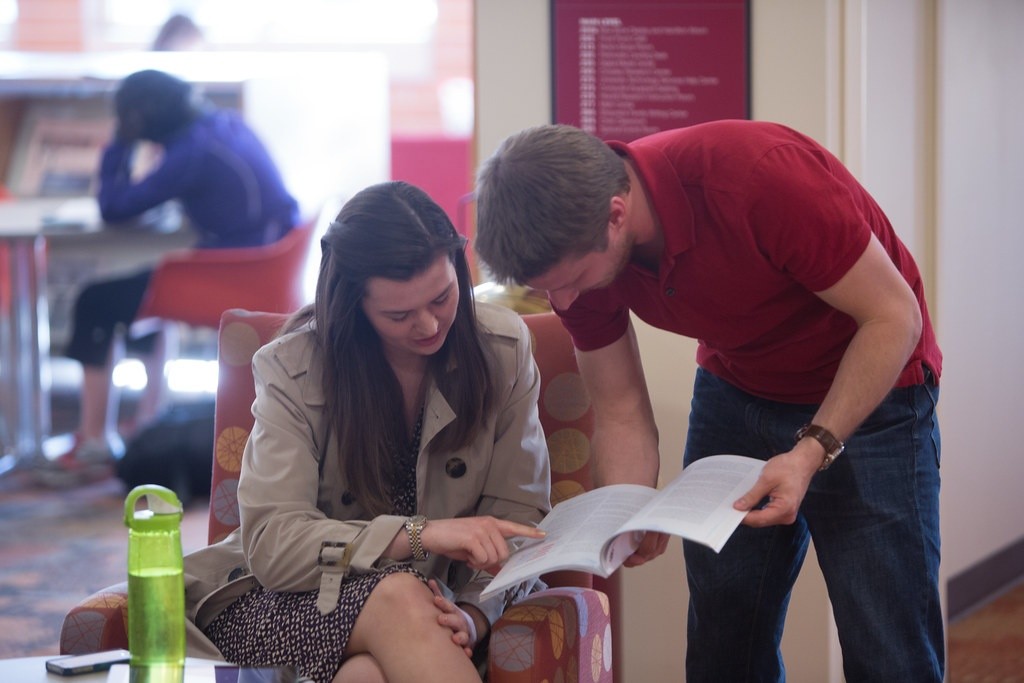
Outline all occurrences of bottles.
[126,484,186,664]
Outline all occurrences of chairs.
[131,197,324,338]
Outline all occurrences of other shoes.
[41,432,124,485]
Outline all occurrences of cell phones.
[46,648,130,676]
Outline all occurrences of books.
[478,454,772,603]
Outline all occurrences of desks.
[1,200,182,478]
[0,654,300,683]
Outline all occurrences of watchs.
[794,424,845,466]
[405,514,430,560]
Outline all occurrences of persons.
[120,180,552,682]
[474,120,945,683]
[40,67,302,482]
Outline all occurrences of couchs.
[59,309,613,683]
